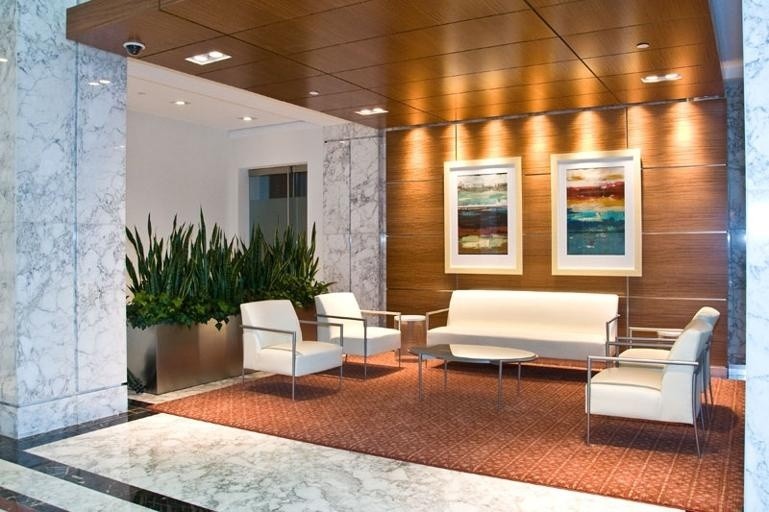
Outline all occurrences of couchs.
[425,289,621,368]
[585,320,713,458]
[314,292,401,379]
[605,306,721,421]
[240,300,344,401]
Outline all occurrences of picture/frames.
[550,147,643,277]
[442,155,523,276]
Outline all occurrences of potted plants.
[127,213,328,394]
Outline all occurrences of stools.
[394,315,426,363]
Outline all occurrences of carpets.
[148,351,746,511]
